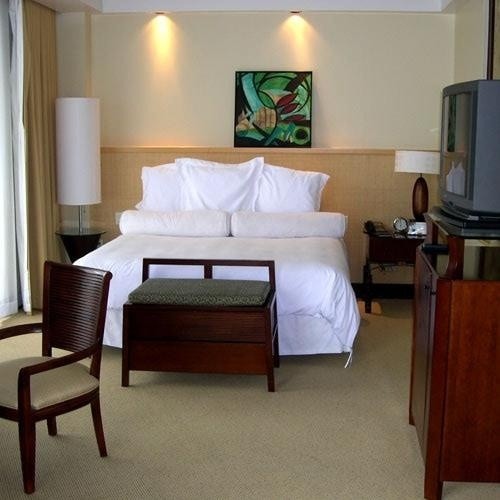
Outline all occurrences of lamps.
[394,150,441,222]
[54,97,108,236]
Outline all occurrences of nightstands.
[54,232,106,264]
[363,230,427,314]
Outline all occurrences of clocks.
[393,216,409,237]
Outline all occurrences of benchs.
[122,258,280,392]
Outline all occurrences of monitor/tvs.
[432,79,500,226]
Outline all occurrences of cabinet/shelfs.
[408,213,500,500]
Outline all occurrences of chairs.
[0,260,113,494]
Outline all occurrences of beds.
[71,234,361,357]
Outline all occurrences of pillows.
[119,156,348,239]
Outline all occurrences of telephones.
[363,220,389,235]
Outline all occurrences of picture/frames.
[234,71,312,148]
